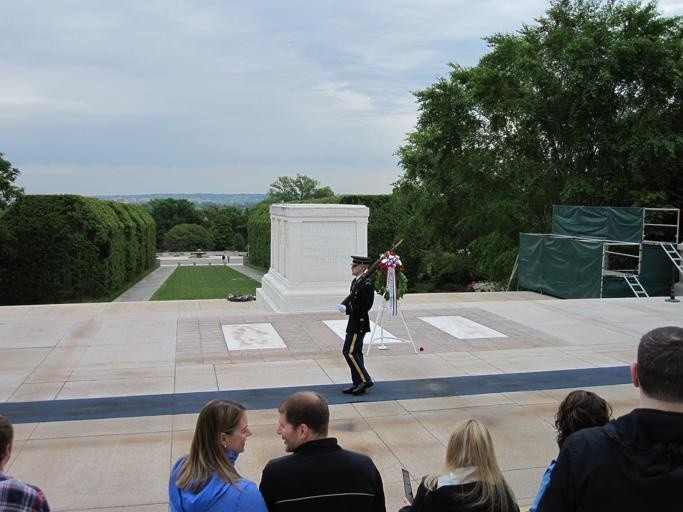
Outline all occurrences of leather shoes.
[342,381,373,395]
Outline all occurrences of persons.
[335,255,374,396]
[166,397,269,511]
[526,389,614,511]
[0,414,49,511]
[257,389,386,512]
[397,417,521,512]
[532,324,682,511]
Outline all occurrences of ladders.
[659,242,683,274]
[624,274,649,298]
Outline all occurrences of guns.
[339,238,404,306]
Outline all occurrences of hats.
[350,256,372,267]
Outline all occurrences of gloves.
[336,304,346,313]
